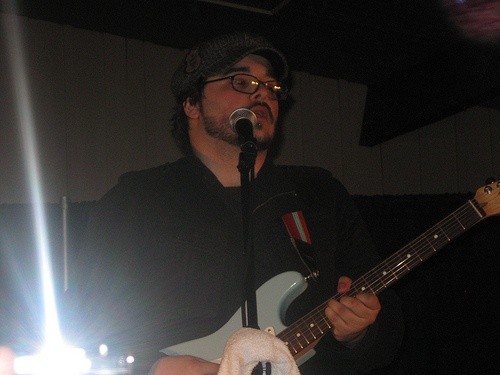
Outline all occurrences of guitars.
[148,181,500,375]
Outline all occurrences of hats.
[169,32,287,97]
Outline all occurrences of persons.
[51,28,406,374]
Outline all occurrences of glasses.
[200,72,288,100]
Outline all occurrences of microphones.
[230,106,257,171]
